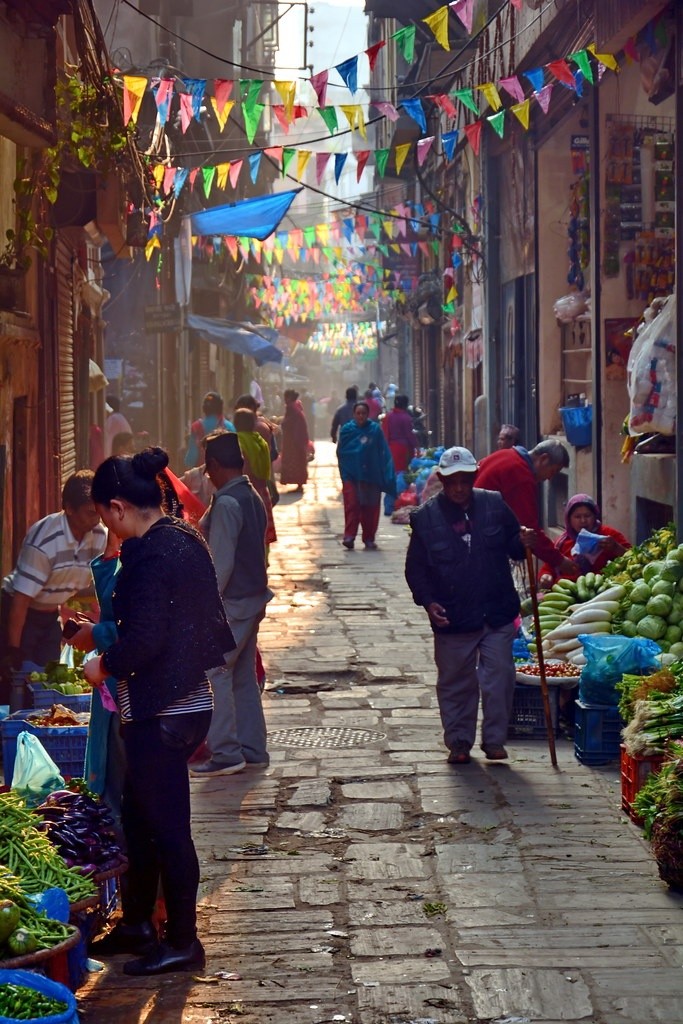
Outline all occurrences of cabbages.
[621,543,683,657]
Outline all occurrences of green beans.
[0,792,100,950]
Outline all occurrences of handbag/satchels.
[261,421,279,461]
[9,730,66,807]
[627,292,677,435]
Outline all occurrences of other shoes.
[343,538,353,548]
[365,540,378,548]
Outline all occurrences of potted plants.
[0,60,151,308]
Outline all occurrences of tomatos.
[26,715,88,726]
[515,662,580,677]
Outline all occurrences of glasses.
[204,463,215,478]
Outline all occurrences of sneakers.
[189,760,245,776]
[481,743,508,759]
[246,760,270,768]
[448,744,470,763]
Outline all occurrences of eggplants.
[35,789,121,875]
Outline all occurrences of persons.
[537,495,632,590]
[404,445,538,766]
[496,425,518,449]
[472,441,580,576]
[0,376,420,777]
[62,446,238,976]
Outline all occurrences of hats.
[437,446,477,476]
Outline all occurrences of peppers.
[65,778,99,803]
[0,983,68,1019]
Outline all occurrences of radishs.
[541,585,626,665]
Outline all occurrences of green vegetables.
[614,658,683,723]
[635,759,683,841]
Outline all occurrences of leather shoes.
[89,917,157,955]
[123,936,206,976]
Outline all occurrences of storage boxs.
[620,745,670,825]
[573,699,628,767]
[507,683,562,740]
[0,709,91,786]
[9,660,92,714]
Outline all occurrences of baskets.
[559,403,592,445]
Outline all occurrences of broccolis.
[608,528,674,583]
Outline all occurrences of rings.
[72,643,77,649]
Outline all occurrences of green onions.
[622,695,683,758]
